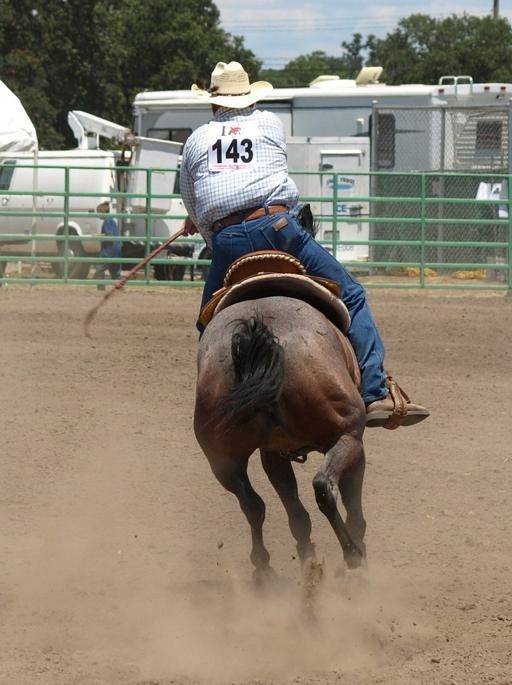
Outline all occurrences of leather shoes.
[366,389,430,427]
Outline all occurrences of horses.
[193,203,367,628]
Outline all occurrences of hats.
[191,61,273,109]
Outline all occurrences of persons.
[179,60,429,428]
[91,202,121,292]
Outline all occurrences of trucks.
[130,64,511,272]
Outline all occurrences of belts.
[211,204,289,233]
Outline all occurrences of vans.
[2,150,193,279]
